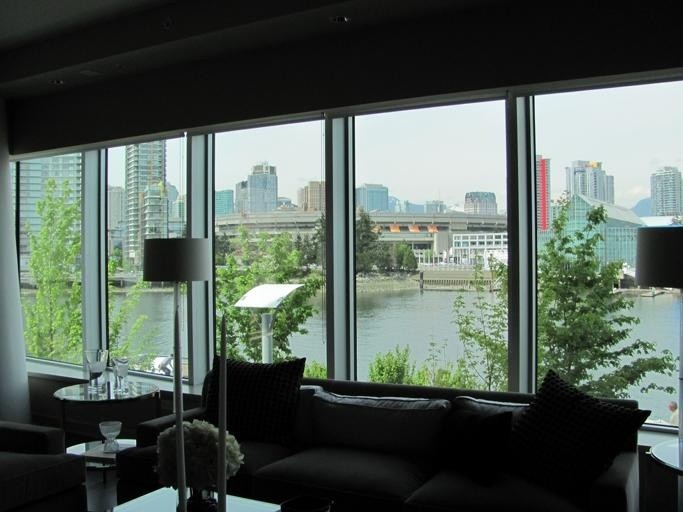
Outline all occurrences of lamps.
[635,225,683,289]
[142,239,213,414]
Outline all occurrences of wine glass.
[100,422,121,453]
[83,350,108,391]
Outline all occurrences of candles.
[216,311,228,511]
[173,305,188,512]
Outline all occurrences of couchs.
[137,379,651,511]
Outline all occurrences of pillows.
[513,369,651,503]
[409,412,514,488]
[212,354,306,450]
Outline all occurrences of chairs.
[0,421,83,512]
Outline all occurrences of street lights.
[231,283,304,363]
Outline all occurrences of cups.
[111,357,129,390]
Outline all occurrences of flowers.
[149,420,259,496]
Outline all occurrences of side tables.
[65,439,137,512]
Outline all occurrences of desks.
[52,383,162,438]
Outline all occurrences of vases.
[177,487,218,512]
